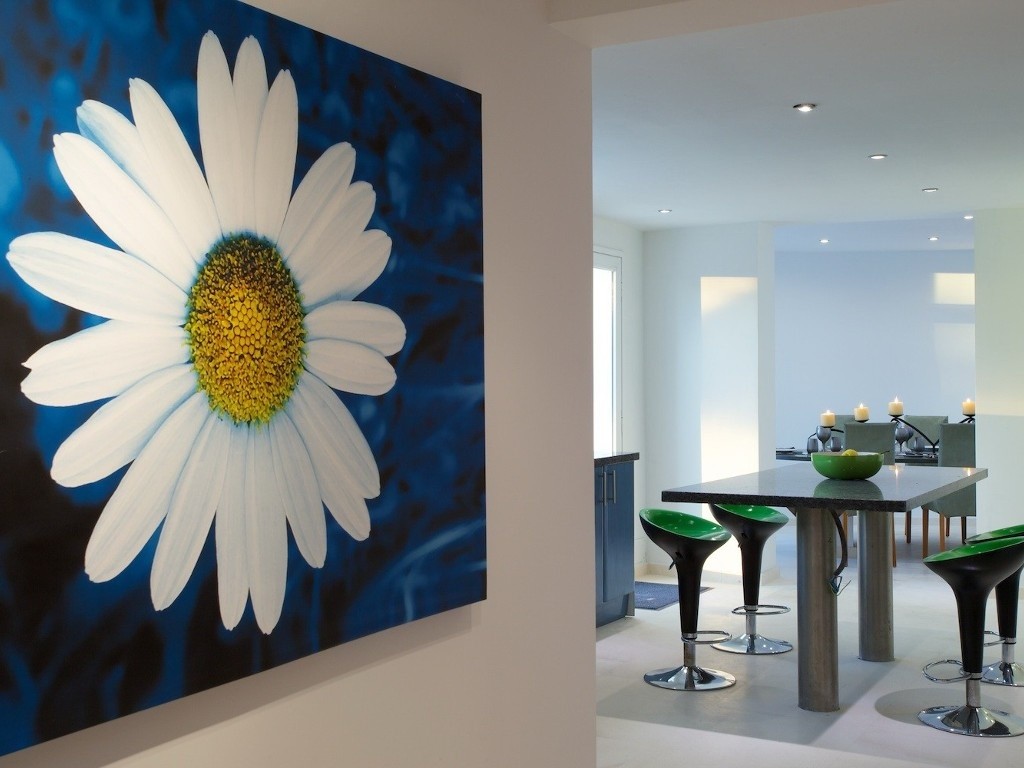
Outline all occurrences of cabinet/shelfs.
[596,461,633,628]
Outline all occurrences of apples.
[841,449,858,456]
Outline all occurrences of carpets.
[634,581,711,612]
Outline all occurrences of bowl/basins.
[810,451,885,481]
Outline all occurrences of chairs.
[841,422,899,568]
[826,415,856,538]
[920,422,975,553]
[904,416,949,543]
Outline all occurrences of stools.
[640,508,736,691]
[918,536,1024,737]
[710,503,792,654]
[958,525,1024,687]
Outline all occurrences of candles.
[821,413,835,425]
[854,407,869,420]
[888,402,903,415]
[963,398,976,414]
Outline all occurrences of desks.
[661,463,989,713]
[775,449,938,559]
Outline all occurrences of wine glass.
[895,427,909,455]
[905,427,916,455]
[816,427,831,452]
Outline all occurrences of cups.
[831,437,841,452]
[807,434,818,453]
[913,436,925,452]
